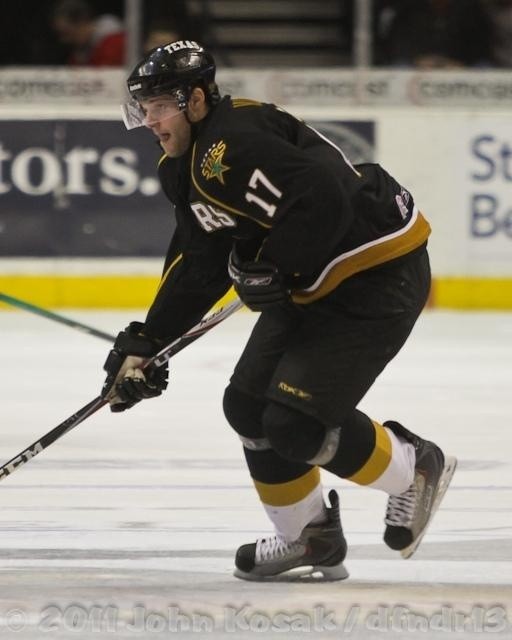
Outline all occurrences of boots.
[382,420,447,552]
[234,489,344,574]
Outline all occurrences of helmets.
[119,38,216,131]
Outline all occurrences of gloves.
[105,323,169,413]
[226,246,291,310]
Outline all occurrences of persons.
[103,36,448,572]
